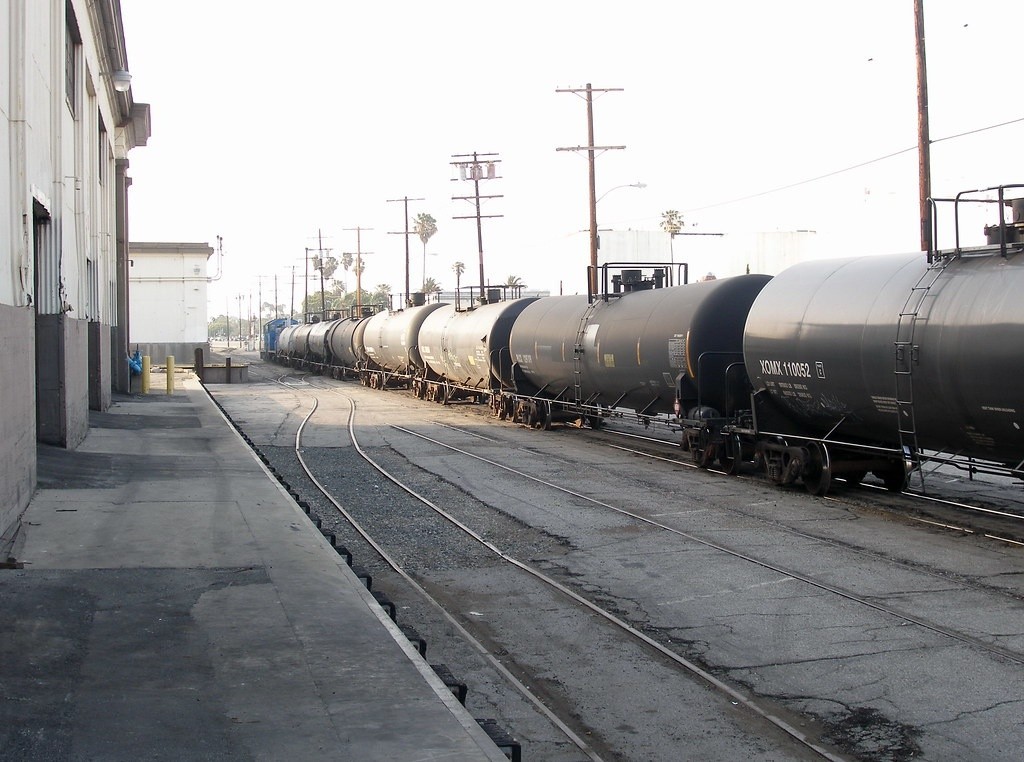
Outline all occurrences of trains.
[260,183,1024,493]
